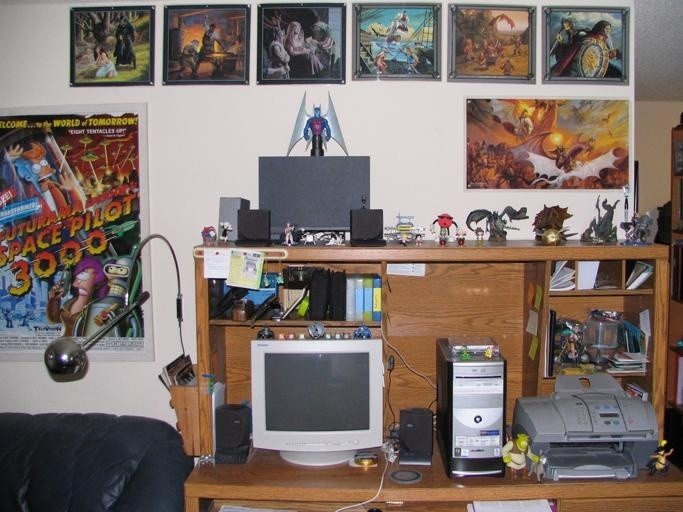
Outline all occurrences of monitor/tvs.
[250,341,384,466]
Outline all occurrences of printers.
[511,375,658,481]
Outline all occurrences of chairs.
[0,411,189,512]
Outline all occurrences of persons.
[194,16,226,73]
[463,36,522,77]
[178,40,199,79]
[554,18,622,78]
[632,211,655,241]
[284,222,294,243]
[269,21,335,77]
[94,45,115,79]
[115,16,137,70]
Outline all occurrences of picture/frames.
[0,100,156,366]
[446,1,538,85]
[255,1,346,85]
[160,1,251,85]
[351,0,442,82]
[69,5,156,87]
[460,91,631,195]
[538,4,631,87]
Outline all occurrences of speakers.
[350,209,386,247]
[399,408,433,466]
[259,156,370,245]
[214,404,251,465]
[235,210,271,246]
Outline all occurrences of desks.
[182,426,680,511]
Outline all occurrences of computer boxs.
[436,338,508,478]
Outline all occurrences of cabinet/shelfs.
[664,125,681,413]
[525,233,672,470]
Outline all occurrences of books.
[549,260,576,291]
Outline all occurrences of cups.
[581,318,618,363]
[231,299,249,321]
